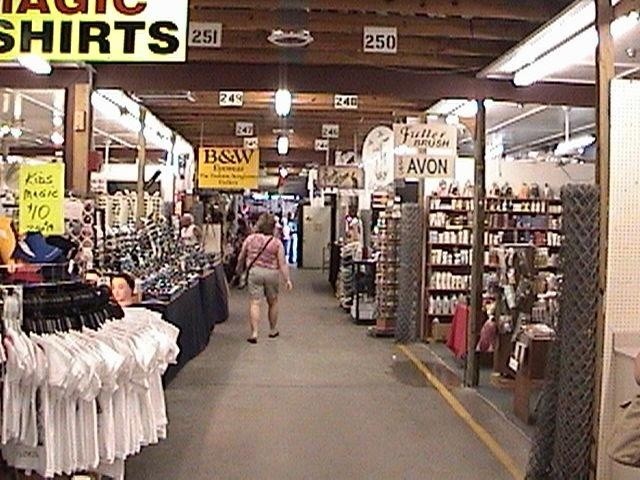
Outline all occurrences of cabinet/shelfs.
[330,190,563,342]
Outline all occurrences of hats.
[15,231,63,262]
[45,235,78,262]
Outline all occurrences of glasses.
[376,210,397,317]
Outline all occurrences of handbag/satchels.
[237,269,248,289]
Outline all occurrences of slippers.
[246,336,257,344]
[269,329,279,338]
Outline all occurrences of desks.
[127,258,224,390]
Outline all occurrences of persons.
[221,208,294,290]
[111,275,135,307]
[228,211,293,344]
[181,211,206,251]
